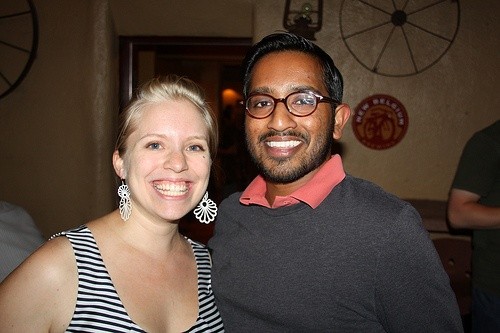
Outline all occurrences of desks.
[395,199,472,317]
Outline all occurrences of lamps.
[281,0,325,45]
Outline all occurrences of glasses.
[240,89,341,119]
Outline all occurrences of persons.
[446,121,500,333]
[0,76,225,333]
[206,32,465,333]
